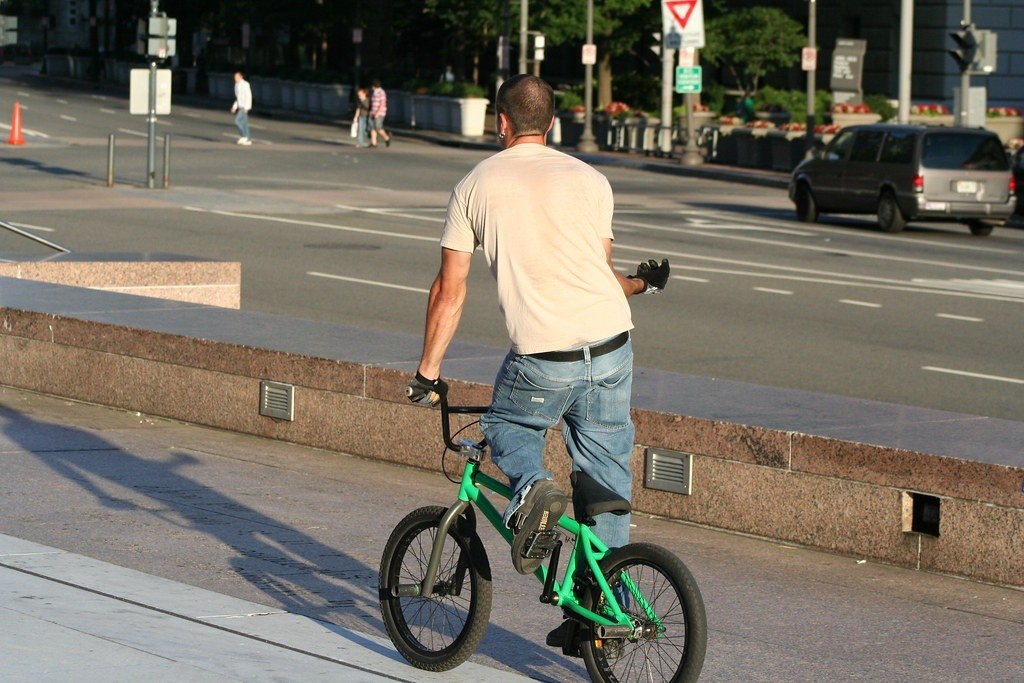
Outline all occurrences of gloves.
[406,370,449,408]
[627,259,671,295]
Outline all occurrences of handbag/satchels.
[350,122,358,137]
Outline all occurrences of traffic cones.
[3,101,27,145]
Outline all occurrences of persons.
[369,80,391,148]
[231,72,252,145]
[440,66,454,83]
[408,74,670,646]
[353,89,371,149]
[740,91,752,110]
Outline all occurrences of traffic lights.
[137,11,177,64]
[949,27,995,79]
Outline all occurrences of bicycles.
[375,386,709,683]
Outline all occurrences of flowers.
[693,103,709,112]
[718,115,740,125]
[605,101,630,115]
[572,105,586,112]
[813,124,842,134]
[911,104,948,115]
[779,122,808,132]
[746,120,775,129]
[986,107,1022,117]
[832,103,871,114]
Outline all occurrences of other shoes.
[367,143,377,149]
[385,137,391,147]
[238,137,252,145]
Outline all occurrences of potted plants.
[553,94,582,145]
[103,44,490,138]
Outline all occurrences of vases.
[573,112,586,123]
[821,112,882,129]
[909,116,955,128]
[768,131,808,173]
[747,129,768,169]
[986,117,1024,146]
[717,125,754,169]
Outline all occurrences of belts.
[526,331,629,361]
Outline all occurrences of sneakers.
[545,621,624,659]
[511,477,569,574]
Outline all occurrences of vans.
[787,123,1016,235]
